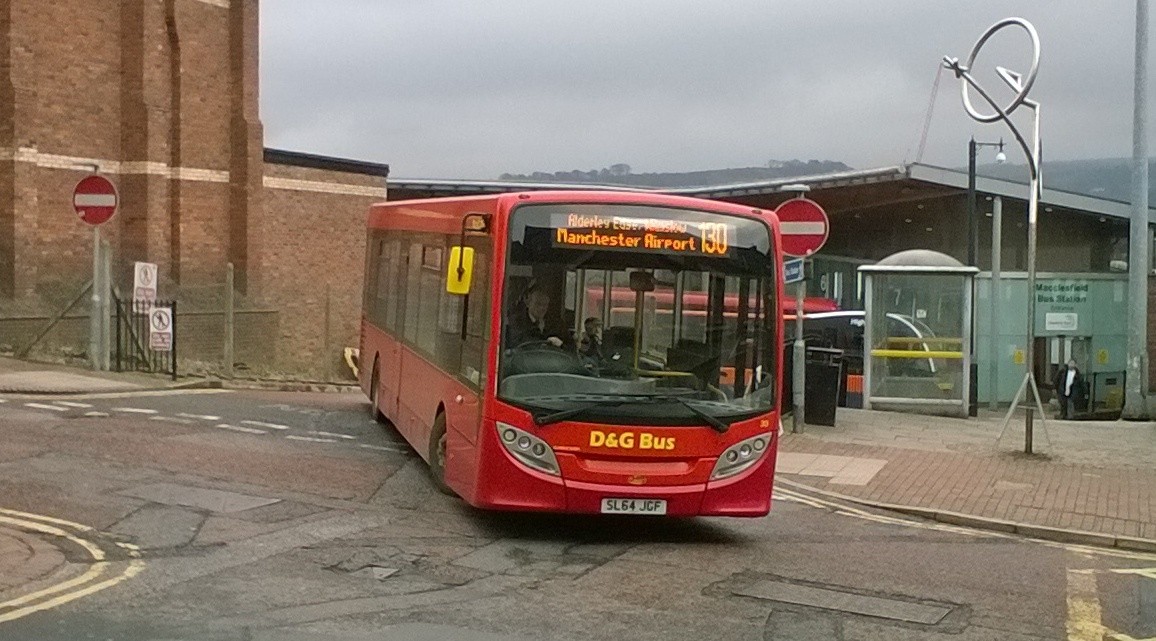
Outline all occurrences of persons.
[1054,359,1084,419]
[508,285,569,351]
[580,318,604,375]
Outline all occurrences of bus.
[581,286,951,399]
[357,191,783,519]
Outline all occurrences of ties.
[535,321,541,331]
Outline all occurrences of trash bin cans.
[804,347,845,426]
[846,374,864,409]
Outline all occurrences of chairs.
[602,325,635,365]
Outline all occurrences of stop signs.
[72,176,119,226]
[769,198,829,258]
